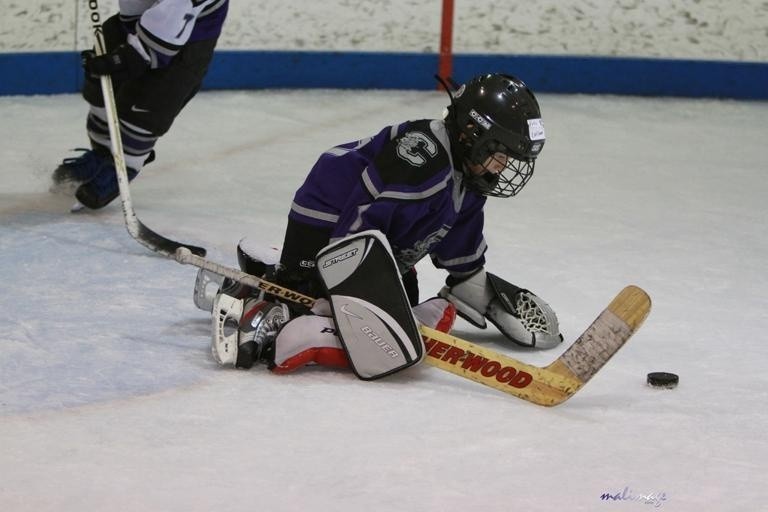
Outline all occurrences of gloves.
[80,43,145,109]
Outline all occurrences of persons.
[217,72,565,383]
[50,0,232,212]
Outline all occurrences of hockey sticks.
[176,248,651,406]
[88,1,206,260]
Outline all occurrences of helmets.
[445,72,547,166]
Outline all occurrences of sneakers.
[236,296,289,370]
[51,148,103,185]
[75,149,158,210]
